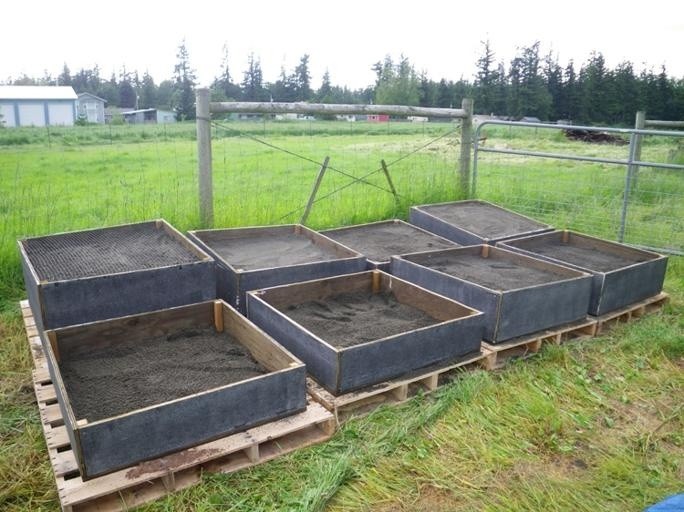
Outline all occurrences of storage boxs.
[321,219,460,271]
[18,218,215,348]
[408,200,555,247]
[183,222,365,315]
[392,245,594,344]
[27,299,308,480]
[496,227,670,319]
[246,266,485,394]
